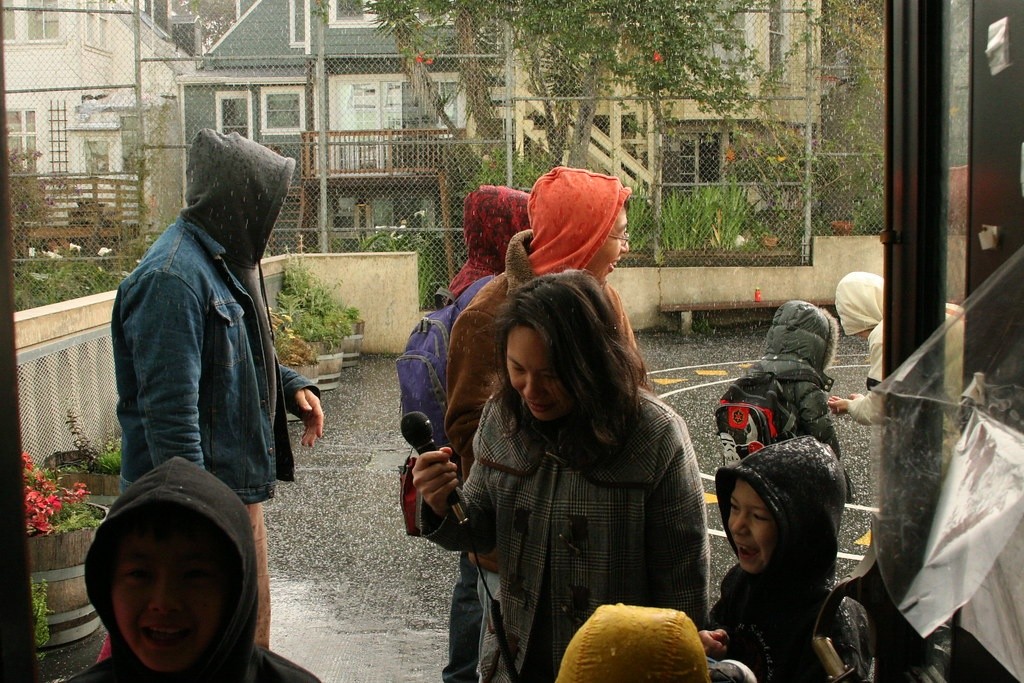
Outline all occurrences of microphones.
[401,412,469,525]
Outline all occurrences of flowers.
[21,450,104,537]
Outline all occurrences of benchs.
[658,297,837,335]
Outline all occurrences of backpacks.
[394,274,498,490]
[712,361,820,469]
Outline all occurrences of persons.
[442,167,632,683]
[57,456,321,683]
[555,603,707,683]
[412,269,709,683]
[110,129,323,651]
[440,185,530,683]
[698,436,876,683]
[744,300,857,505]
[826,270,966,489]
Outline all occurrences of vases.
[25,502,110,647]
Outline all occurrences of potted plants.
[41,403,124,496]
[268,253,364,421]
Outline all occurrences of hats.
[551,603,713,683]
